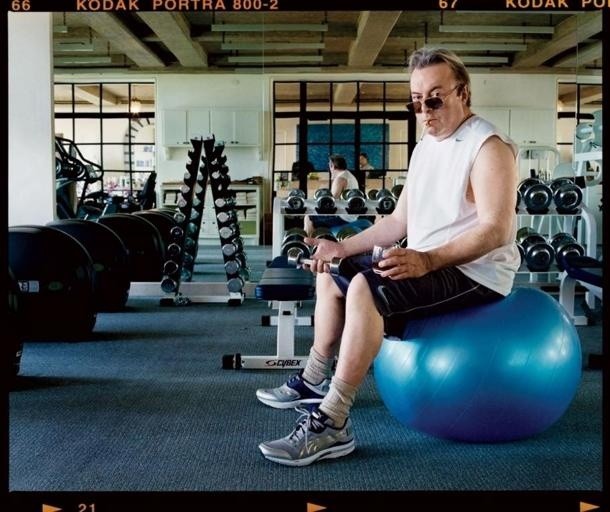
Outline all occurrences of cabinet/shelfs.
[161,182,262,249]
[160,109,261,147]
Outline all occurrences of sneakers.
[256,412,357,467]
[254,370,333,409]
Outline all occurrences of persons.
[255,41,524,467]
[303,153,360,236]
[359,152,374,170]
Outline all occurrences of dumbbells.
[520,232,585,271]
[281,226,358,275]
[287,178,583,212]
[161,134,249,292]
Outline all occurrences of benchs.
[220,266,315,370]
[559,266,603,328]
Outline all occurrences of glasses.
[405,83,460,114]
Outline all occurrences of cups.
[372,242,399,278]
